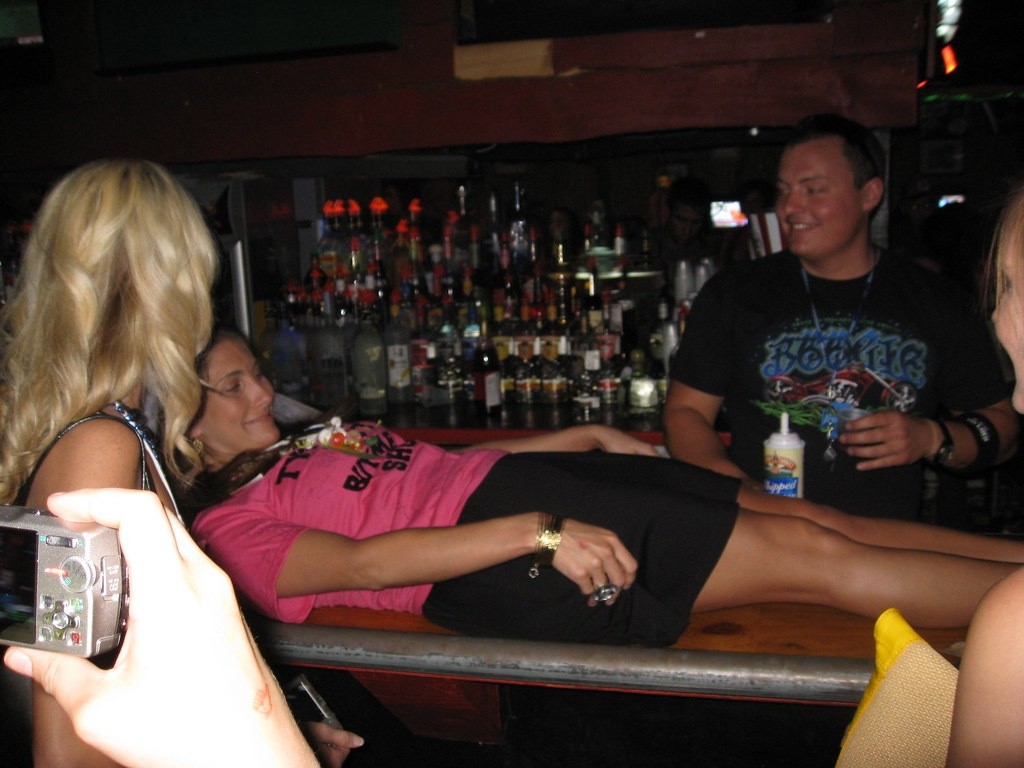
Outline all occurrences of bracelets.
[536,511,566,565]
[950,412,1000,476]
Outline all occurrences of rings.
[591,583,617,601]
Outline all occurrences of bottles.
[761,412,806,506]
[249,179,723,420]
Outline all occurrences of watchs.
[921,417,955,471]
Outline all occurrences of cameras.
[285,674,344,749]
[0,503,131,658]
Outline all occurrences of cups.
[834,408,873,453]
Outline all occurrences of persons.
[5,488,319,768]
[1,162,365,768]
[946,197,1024,768]
[662,116,1017,531]
[163,332,1024,647]
